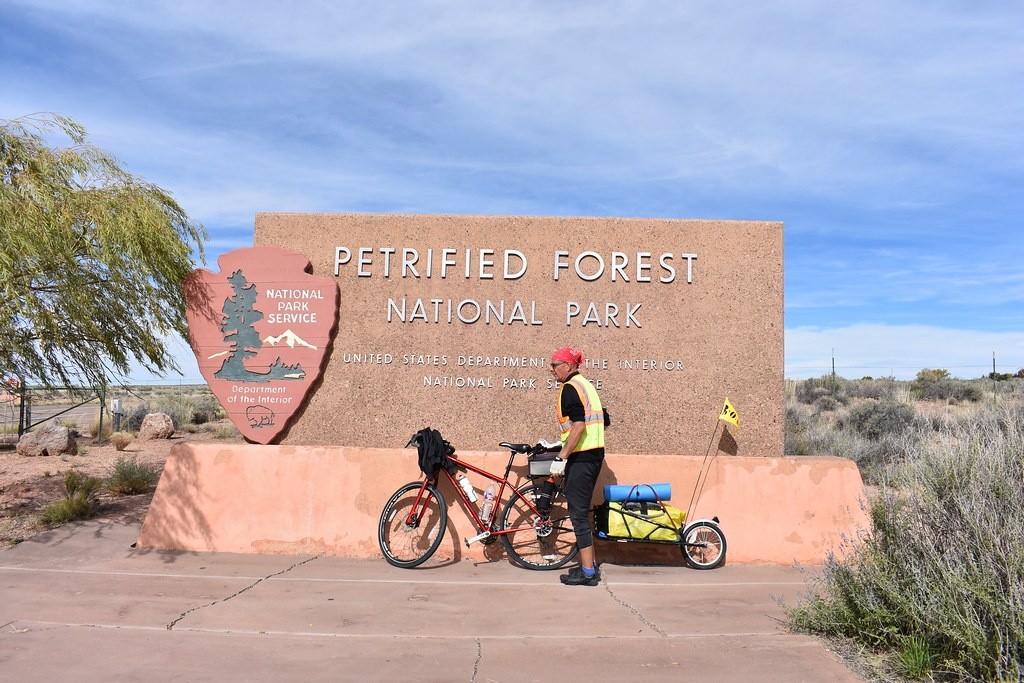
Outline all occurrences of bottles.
[458,474,478,503]
[546,474,554,484]
[479,482,495,521]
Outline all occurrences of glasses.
[550,361,564,369]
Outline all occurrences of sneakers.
[560,566,598,586]
[568,564,601,581]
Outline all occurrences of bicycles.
[378,433,580,571]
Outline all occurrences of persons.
[549,348,610,586]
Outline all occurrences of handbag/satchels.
[527,452,560,476]
[594,501,687,541]
